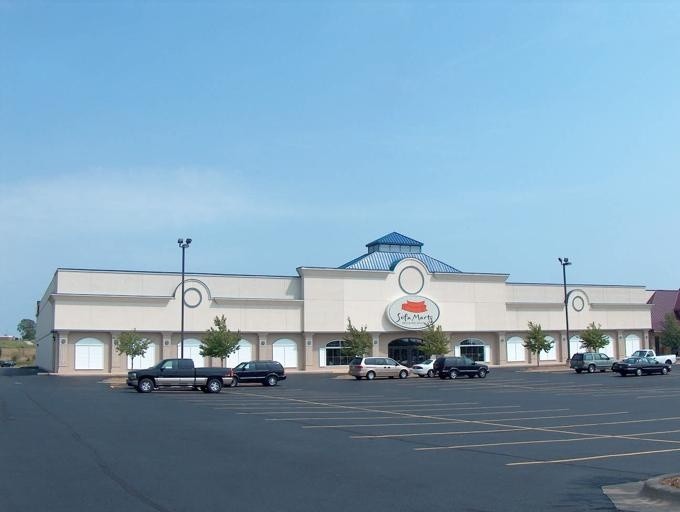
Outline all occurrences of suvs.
[433,356,491,380]
[569,352,618,372]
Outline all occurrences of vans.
[228,359,287,388]
[347,355,411,380]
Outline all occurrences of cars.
[0,359,16,368]
[612,357,672,377]
[411,357,439,378]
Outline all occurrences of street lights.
[556,256,573,366]
[178,237,193,359]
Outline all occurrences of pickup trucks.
[125,357,235,395]
[629,349,677,365]
